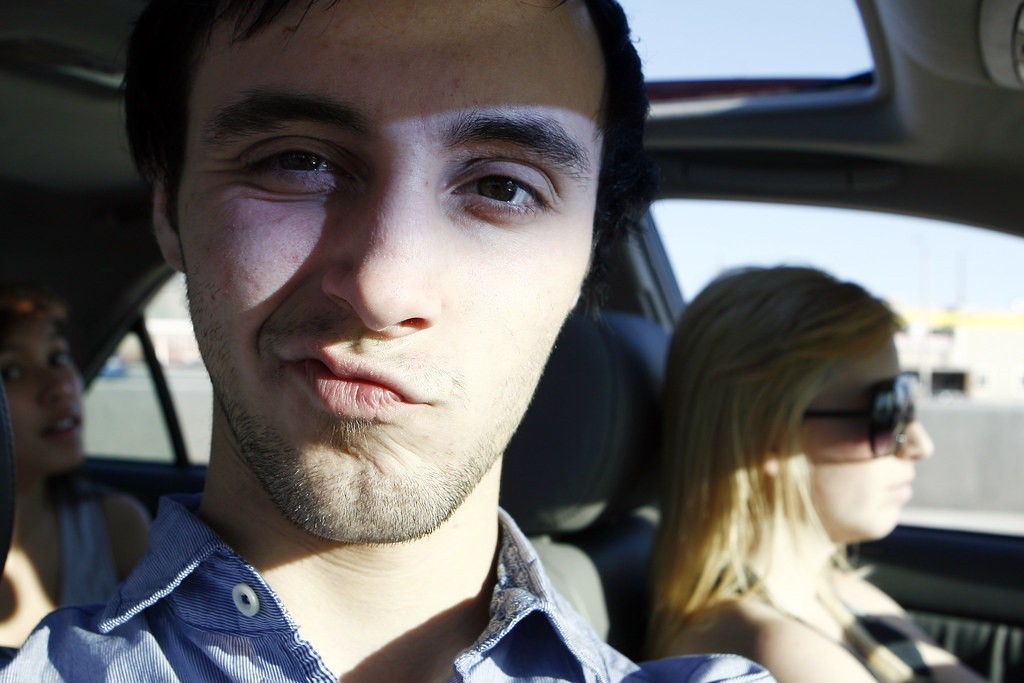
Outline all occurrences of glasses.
[802,372,921,457]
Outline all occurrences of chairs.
[497,310,678,666]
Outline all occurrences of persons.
[646,264,994,682]
[2,0,776,683]
[0,281,153,670]
